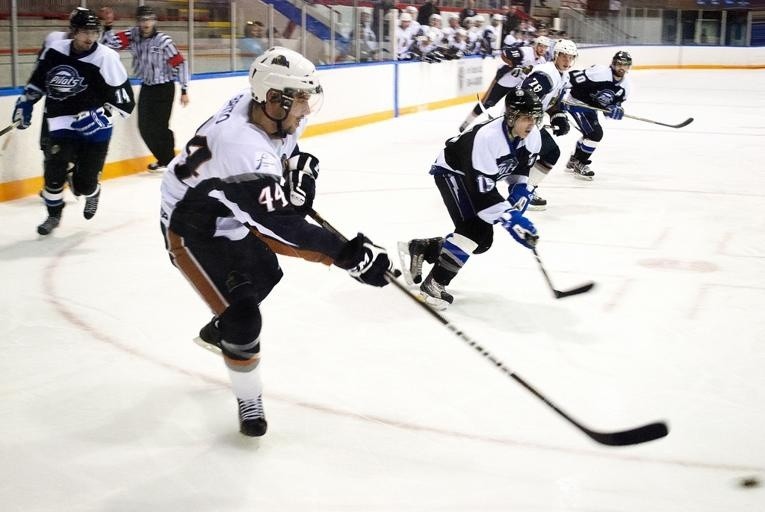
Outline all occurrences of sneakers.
[459,117,471,133]
[408,237,428,284]
[83,184,103,220]
[527,189,548,207]
[565,155,595,177]
[236,392,268,438]
[199,312,223,351]
[146,159,169,172]
[37,214,62,238]
[419,269,454,305]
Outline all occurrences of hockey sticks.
[476,93,561,131]
[562,100,694,128]
[528,237,595,301]
[307,208,667,446]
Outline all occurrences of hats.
[134,5,158,21]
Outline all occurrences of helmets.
[247,45,324,106]
[552,38,580,67]
[534,35,551,51]
[66,6,105,31]
[609,50,634,73]
[360,5,537,43]
[506,88,544,133]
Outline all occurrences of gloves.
[281,151,320,220]
[12,84,44,130]
[495,206,541,249]
[549,110,570,137]
[70,106,113,137]
[333,232,403,288]
[507,182,530,216]
[605,104,624,120]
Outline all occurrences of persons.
[102,6,190,169]
[405,88,543,305]
[162,47,401,436]
[12,7,136,236]
[238,21,281,71]
